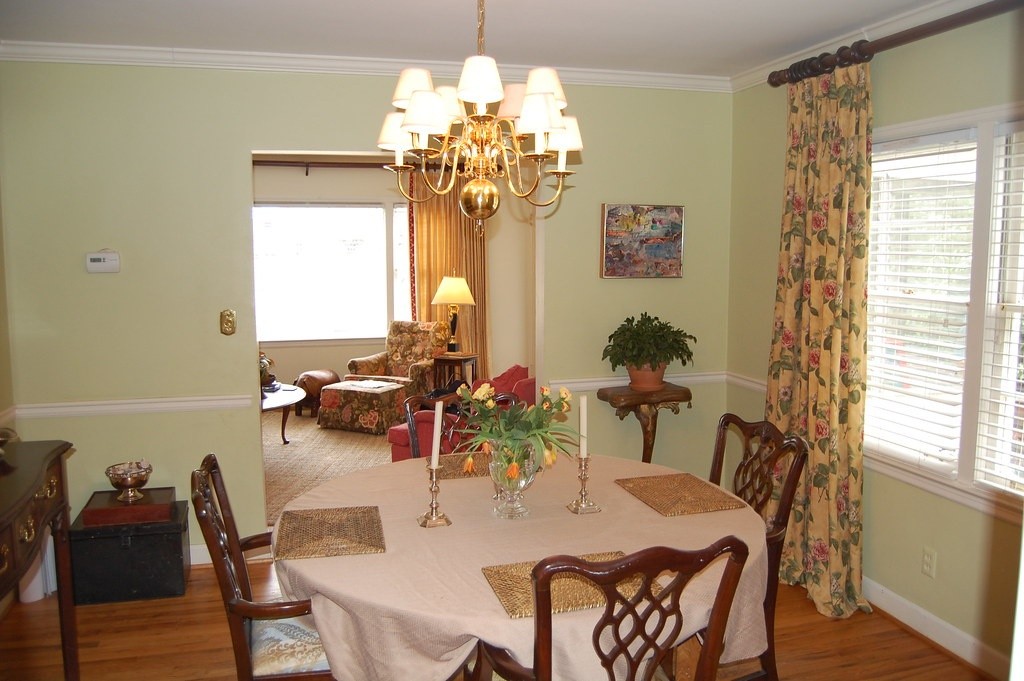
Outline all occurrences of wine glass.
[105,462,153,502]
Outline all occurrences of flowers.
[449,383,582,479]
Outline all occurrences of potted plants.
[602,312,697,391]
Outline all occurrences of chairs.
[404,379,519,458]
[708,413,808,681]
[464,535,748,681]
[191,453,333,681]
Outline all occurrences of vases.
[487,439,536,518]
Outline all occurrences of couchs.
[344,320,450,415]
[316,380,407,435]
[387,365,535,463]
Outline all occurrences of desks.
[270,453,769,681]
[262,384,306,444]
[597,381,692,463]
[430,355,478,389]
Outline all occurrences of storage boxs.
[69,500,188,605]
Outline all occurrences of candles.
[579,395,588,457]
[431,401,444,468]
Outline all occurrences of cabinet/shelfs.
[0,439,80,681]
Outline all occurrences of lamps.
[431,276,476,357]
[376,0,583,236]
